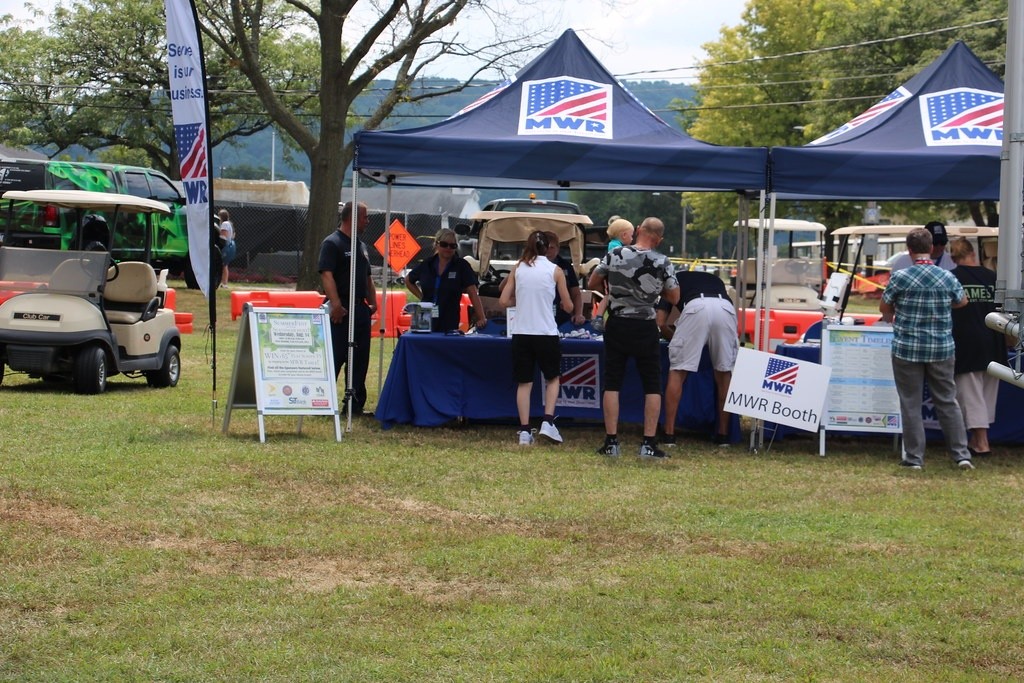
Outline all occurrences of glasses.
[436,241,458,249]
[933,241,946,246]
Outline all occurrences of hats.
[925,221,947,241]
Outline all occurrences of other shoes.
[341,409,374,417]
[898,460,922,470]
[717,431,731,446]
[218,283,228,290]
[957,459,976,469]
[659,430,676,447]
[591,318,605,334]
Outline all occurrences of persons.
[587,215,741,459]
[499,231,585,327]
[878,221,1018,472]
[499,229,574,446]
[406,228,488,333]
[317,200,378,417]
[219,209,236,288]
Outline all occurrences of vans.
[0,158,222,289]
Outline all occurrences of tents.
[344,27,1006,448]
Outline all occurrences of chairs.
[50,259,99,300]
[100,261,157,323]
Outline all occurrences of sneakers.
[638,442,671,458]
[596,438,620,457]
[516,428,537,445]
[539,421,563,442]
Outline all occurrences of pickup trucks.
[454,198,639,286]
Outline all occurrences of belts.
[684,293,733,306]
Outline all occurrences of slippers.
[968,448,992,456]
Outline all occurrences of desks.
[777,344,1024,439]
[397,327,741,448]
